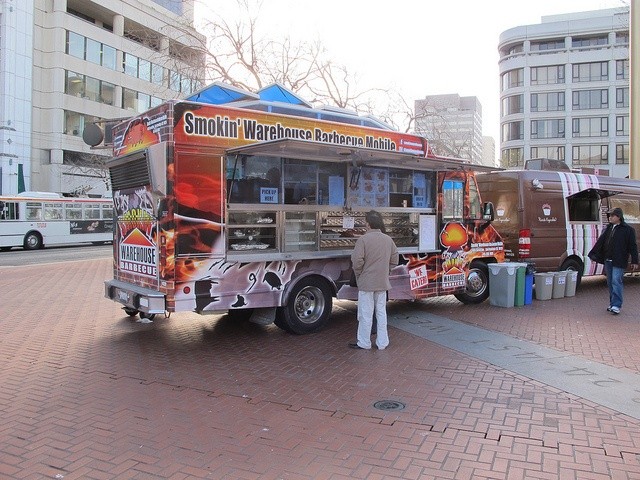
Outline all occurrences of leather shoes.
[349,343,362,349]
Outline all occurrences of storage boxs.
[523,262,535,305]
[550,271,567,298]
[563,269,578,297]
[509,262,527,306]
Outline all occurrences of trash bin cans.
[524,264,536,305]
[565,271,578,297]
[547,271,567,299]
[514,264,527,306]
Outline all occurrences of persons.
[348,210,400,351]
[587,206,640,314]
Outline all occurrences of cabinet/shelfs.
[283,158,438,210]
[227,207,420,250]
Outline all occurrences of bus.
[0,191,114,252]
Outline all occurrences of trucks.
[474,158,640,289]
[103,98,505,334]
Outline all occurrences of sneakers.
[611,306,620,314]
[607,306,611,311]
